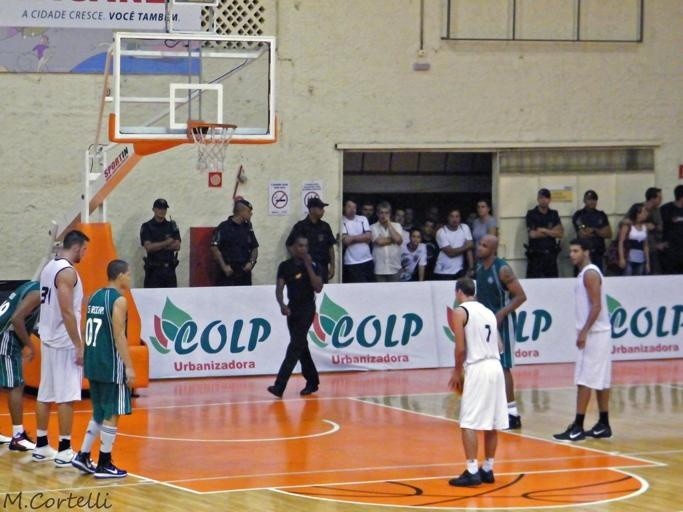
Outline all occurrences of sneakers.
[508,414,522,429]
[584,422,613,440]
[94,462,127,478]
[9,438,35,451]
[54,452,76,467]
[307,198,329,207]
[0,433,12,445]
[32,448,56,462]
[554,427,584,441]
[449,469,480,485]
[300,381,318,396]
[479,467,495,483]
[71,454,95,474]
[267,386,283,397]
[155,199,170,209]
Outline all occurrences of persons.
[72,260,136,478]
[370,202,403,282]
[471,200,497,238]
[400,227,427,281]
[31,230,93,467]
[618,203,651,276]
[360,202,375,226]
[420,220,439,272]
[267,232,324,400]
[210,200,259,286]
[552,237,613,443]
[447,277,509,487]
[0,281,41,450]
[285,198,337,284]
[433,209,475,279]
[525,188,563,278]
[467,234,527,430]
[660,185,683,274]
[342,199,374,283]
[139,198,181,288]
[393,206,438,229]
[572,190,613,277]
[618,187,664,275]
